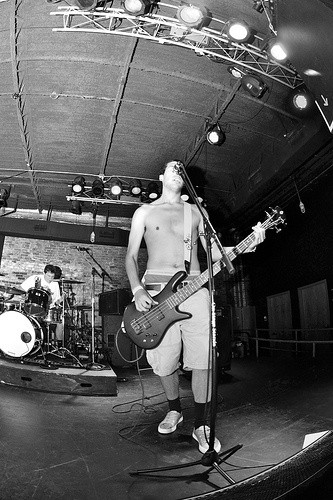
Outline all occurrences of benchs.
[254,328,333,359]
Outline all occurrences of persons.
[20,265,64,356]
[125,159,265,454]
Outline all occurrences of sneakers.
[192,425,222,454]
[157,410,183,435]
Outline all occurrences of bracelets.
[132,285,143,295]
[245,247,256,252]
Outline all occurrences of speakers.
[99,289,130,315]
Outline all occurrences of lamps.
[92,180,104,196]
[293,180,306,213]
[178,2,210,28]
[253,3,267,13]
[0,188,9,204]
[75,0,113,11]
[90,218,96,243]
[293,94,314,112]
[132,179,143,196]
[303,69,322,93]
[110,181,122,196]
[71,177,86,193]
[206,128,226,147]
[268,38,289,63]
[146,182,159,199]
[241,74,268,99]
[221,17,252,43]
[120,0,159,16]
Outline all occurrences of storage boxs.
[98,290,126,316]
[103,315,129,368]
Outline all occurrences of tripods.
[129,170,243,484]
[40,276,105,367]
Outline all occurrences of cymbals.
[0,273,5,276]
[0,285,26,295]
[69,306,92,309]
[52,280,86,284]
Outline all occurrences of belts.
[145,281,189,291]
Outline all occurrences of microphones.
[92,267,102,278]
[174,162,182,171]
[72,246,87,251]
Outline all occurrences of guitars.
[120,205,287,350]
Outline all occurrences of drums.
[24,287,52,317]
[3,301,20,312]
[43,309,63,324]
[0,311,44,358]
